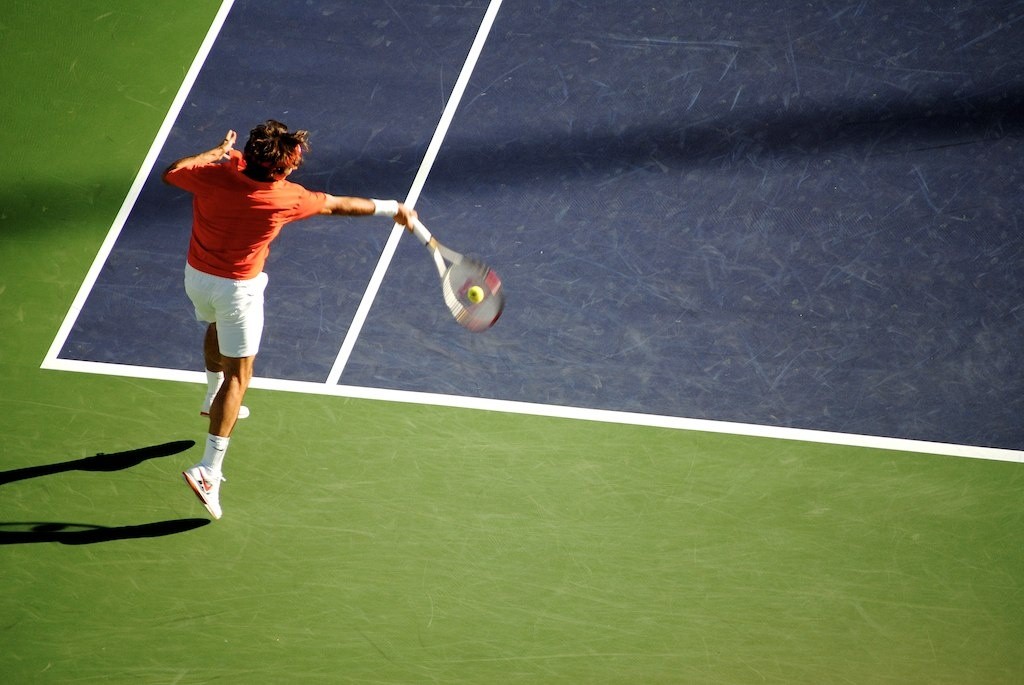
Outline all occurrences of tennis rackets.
[410,215,506,333]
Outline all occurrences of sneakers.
[200,393,250,419]
[182,463,226,519]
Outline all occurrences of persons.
[164,119,418,520]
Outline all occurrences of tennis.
[467,285,484,305]
